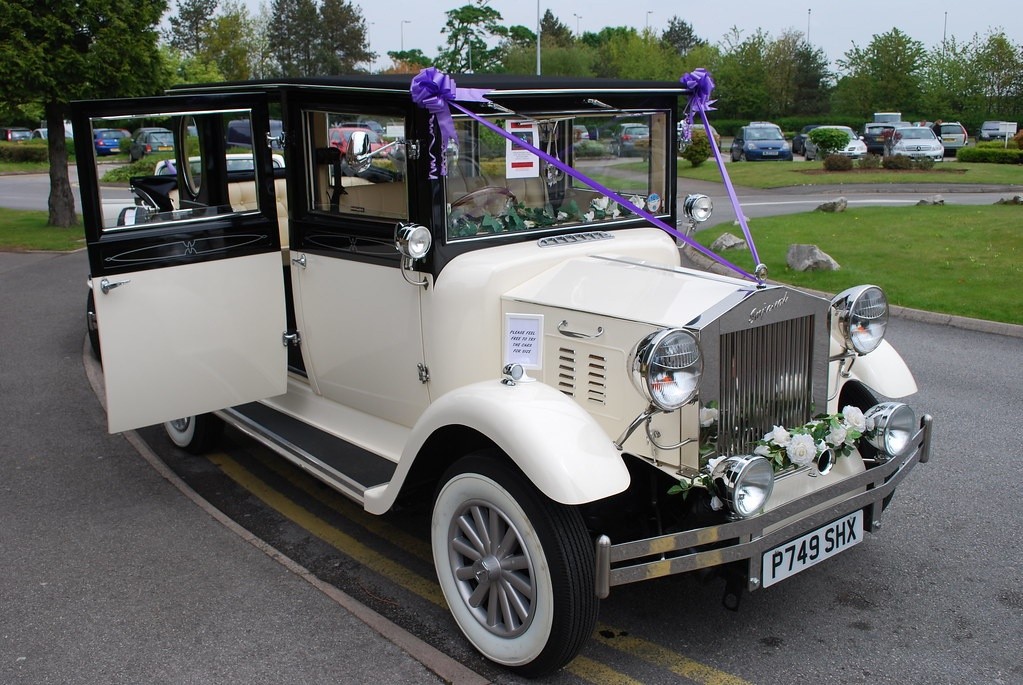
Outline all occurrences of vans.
[226,120,283,152]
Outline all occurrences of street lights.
[646,12,653,29]
[368,22,375,74]
[808,9,810,44]
[401,20,412,51]
[574,14,582,35]
[943,12,947,55]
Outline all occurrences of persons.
[929,119,942,143]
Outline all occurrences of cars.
[792,124,821,154]
[730,121,793,162]
[881,127,945,162]
[70,70,933,678]
[976,121,1015,142]
[0,127,72,142]
[93,128,173,162]
[806,125,867,161]
[690,124,722,153]
[864,121,933,153]
[154,154,286,187]
[330,121,405,162]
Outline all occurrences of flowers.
[445,194,649,239]
[668,405,873,507]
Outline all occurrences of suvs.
[930,122,968,147]
[609,123,649,157]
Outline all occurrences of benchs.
[163,173,377,265]
[330,176,545,218]
